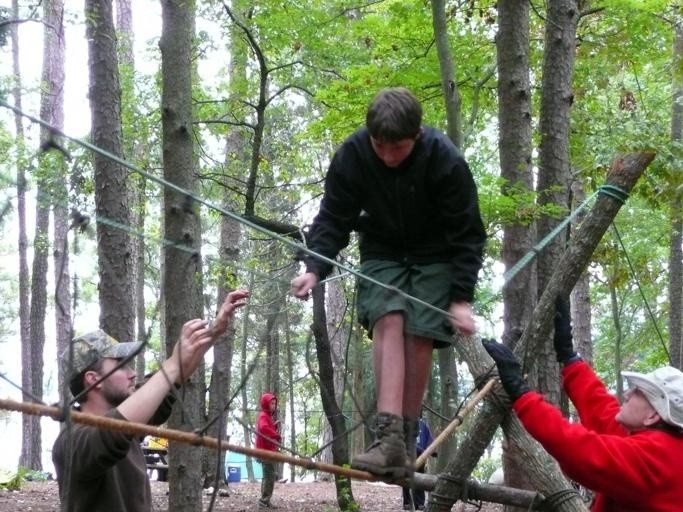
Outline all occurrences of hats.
[620,365,683,429]
[59,328,145,384]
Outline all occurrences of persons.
[51,290,250,512]
[255,392,283,509]
[290,88,486,475]
[398,403,435,509]
[483,290,683,512]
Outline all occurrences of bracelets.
[567,352,581,362]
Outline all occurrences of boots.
[352,414,419,478]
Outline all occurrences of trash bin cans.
[228,467,240,482]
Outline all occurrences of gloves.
[553,295,577,362]
[481,338,530,403]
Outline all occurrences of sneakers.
[258,502,281,512]
